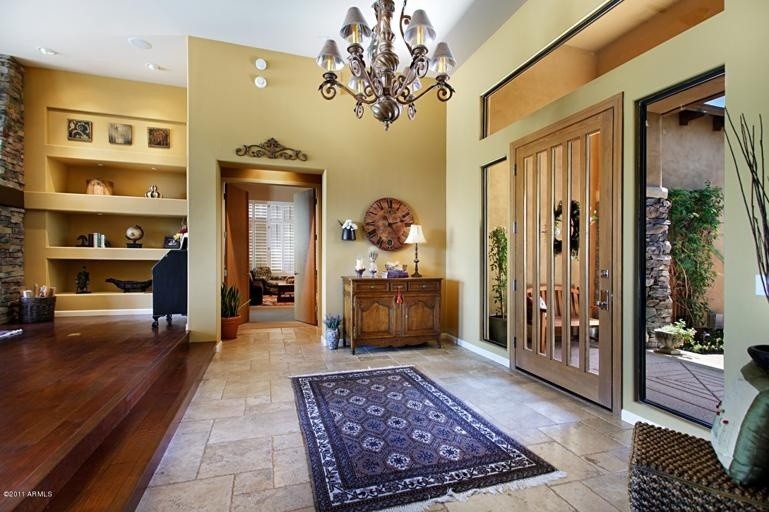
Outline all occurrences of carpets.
[290,365,567,512]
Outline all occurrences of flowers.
[341,219,358,230]
[323,313,342,329]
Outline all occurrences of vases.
[326,328,340,350]
[342,229,357,241]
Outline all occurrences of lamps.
[404,225,426,278]
[316,0,459,130]
[368,245,378,277]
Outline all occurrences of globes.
[125,224,144,248]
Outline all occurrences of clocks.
[364,198,415,250]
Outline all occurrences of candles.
[356,256,364,271]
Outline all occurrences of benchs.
[527,284,599,353]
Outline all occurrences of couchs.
[249,266,295,305]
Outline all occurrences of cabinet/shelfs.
[24,67,185,311]
[342,276,443,355]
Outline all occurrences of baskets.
[19,296,56,323]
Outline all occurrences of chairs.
[628,361,769,512]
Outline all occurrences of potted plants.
[220,280,252,340]
[486,224,507,343]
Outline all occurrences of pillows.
[710,361,769,486]
